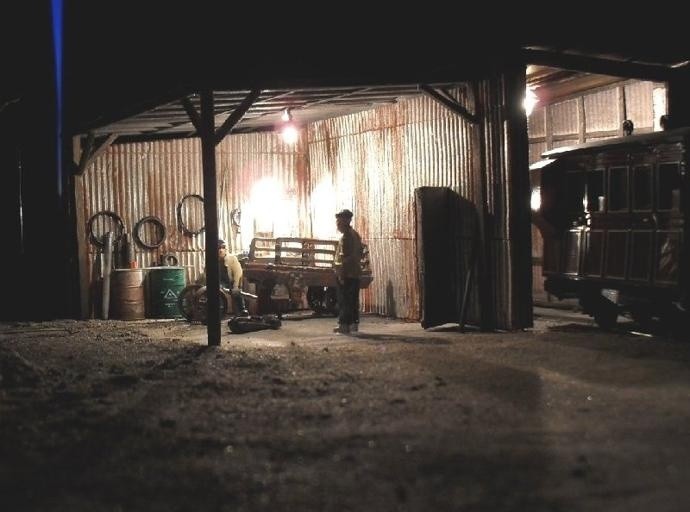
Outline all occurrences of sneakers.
[240,309,248,317]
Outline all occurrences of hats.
[336,210,353,223]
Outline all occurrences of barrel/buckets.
[150,270,186,318]
[112,271,145,319]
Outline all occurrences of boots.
[333,321,358,334]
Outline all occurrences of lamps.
[527,81,540,103]
[281,105,301,126]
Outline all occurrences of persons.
[188,238,247,319]
[333,209,361,335]
